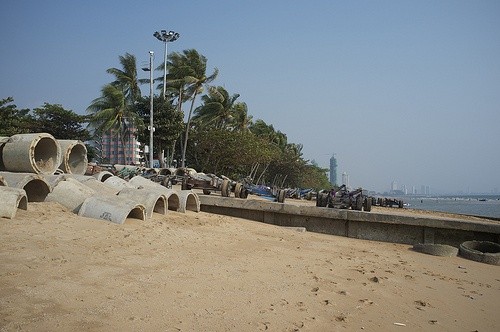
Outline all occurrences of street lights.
[153,29,181,101]
